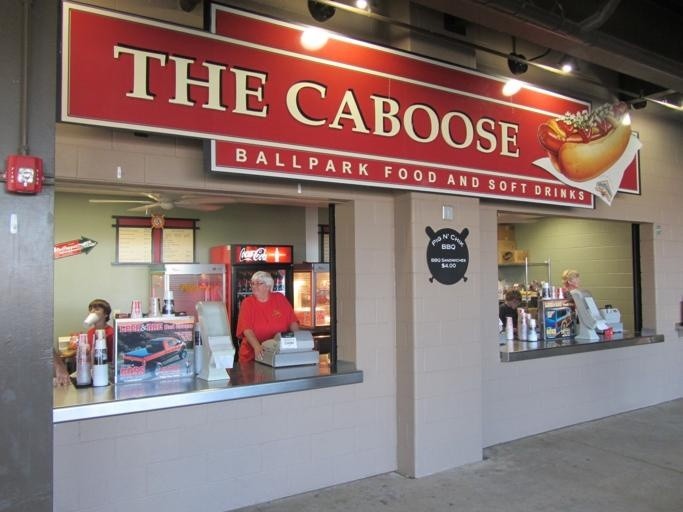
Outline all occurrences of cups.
[504,316,513,339]
[146,297,162,318]
[82,312,98,328]
[129,299,143,318]
[162,291,176,317]
[75,333,91,387]
[193,322,202,375]
[541,282,566,300]
[93,329,109,386]
[516,309,536,342]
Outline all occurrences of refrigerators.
[208,242,294,357]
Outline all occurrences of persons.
[234,270,299,364]
[499,290,521,326]
[559,270,579,298]
[82,299,113,358]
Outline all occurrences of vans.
[546,307,575,339]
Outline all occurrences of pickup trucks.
[123,336,186,375]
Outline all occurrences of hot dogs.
[537,100,633,183]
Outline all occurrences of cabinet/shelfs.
[498,255,553,306]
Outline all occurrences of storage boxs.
[497,240,528,264]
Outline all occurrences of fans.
[82,189,237,218]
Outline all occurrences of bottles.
[236,293,251,315]
[231,268,283,292]
